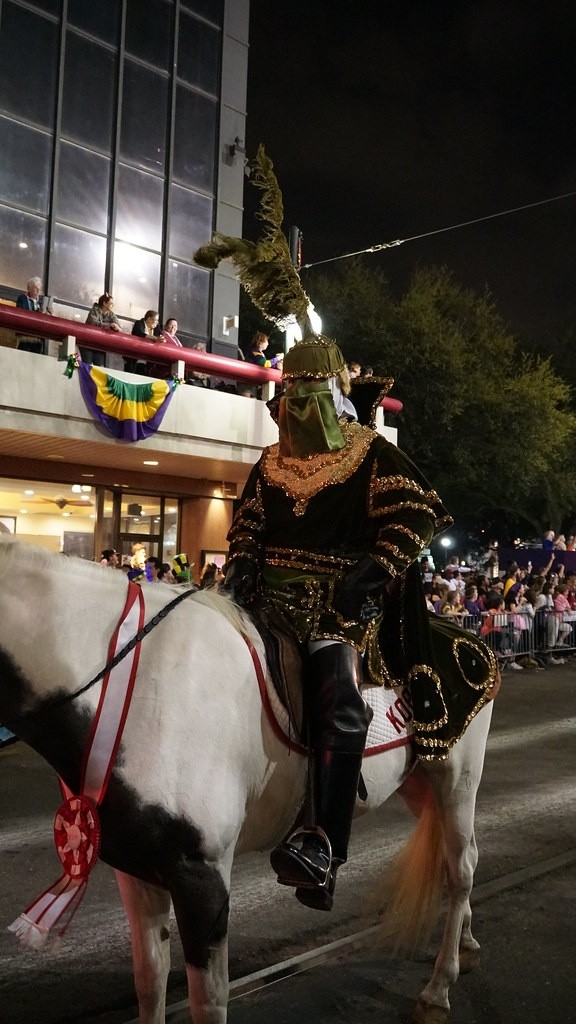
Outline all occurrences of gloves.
[224,558,258,606]
[334,555,395,622]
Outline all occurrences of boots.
[269,644,373,911]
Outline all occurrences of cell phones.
[528,561,531,565]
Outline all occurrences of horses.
[0,530,496,1024]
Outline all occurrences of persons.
[78,292,123,368]
[16,276,53,355]
[236,331,284,398]
[123,310,183,379]
[344,360,373,378]
[186,342,213,388]
[221,332,453,913]
[98,548,226,594]
[419,531,576,671]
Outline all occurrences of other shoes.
[549,657,564,665]
[507,662,523,670]
[557,642,570,648]
